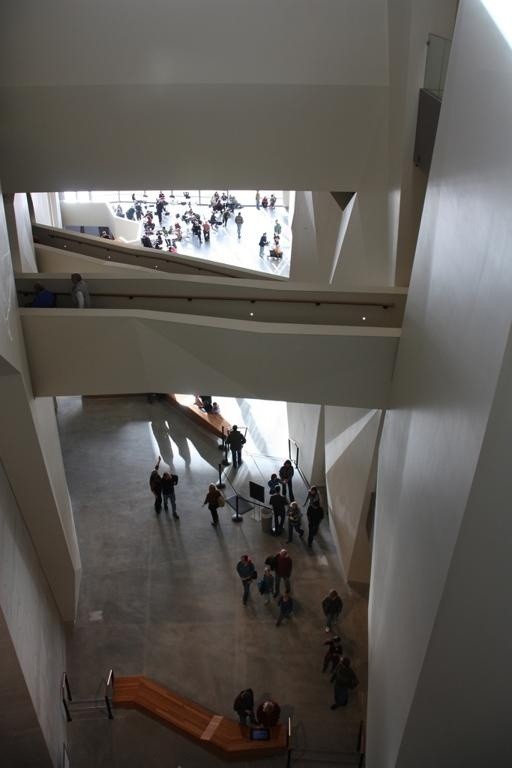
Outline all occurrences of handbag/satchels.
[252,571,257,579]
[217,496,225,507]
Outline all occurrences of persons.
[236,555,255,607]
[195,396,220,414]
[323,590,343,633]
[323,636,357,711]
[272,549,292,597]
[150,457,179,521]
[269,459,324,549]
[276,590,293,627]
[202,484,222,528]
[225,425,247,469]
[261,568,274,605]
[31,282,57,308]
[233,688,280,734]
[100,191,288,262]
[69,272,92,310]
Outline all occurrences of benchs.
[111,675,295,759]
[168,394,237,438]
